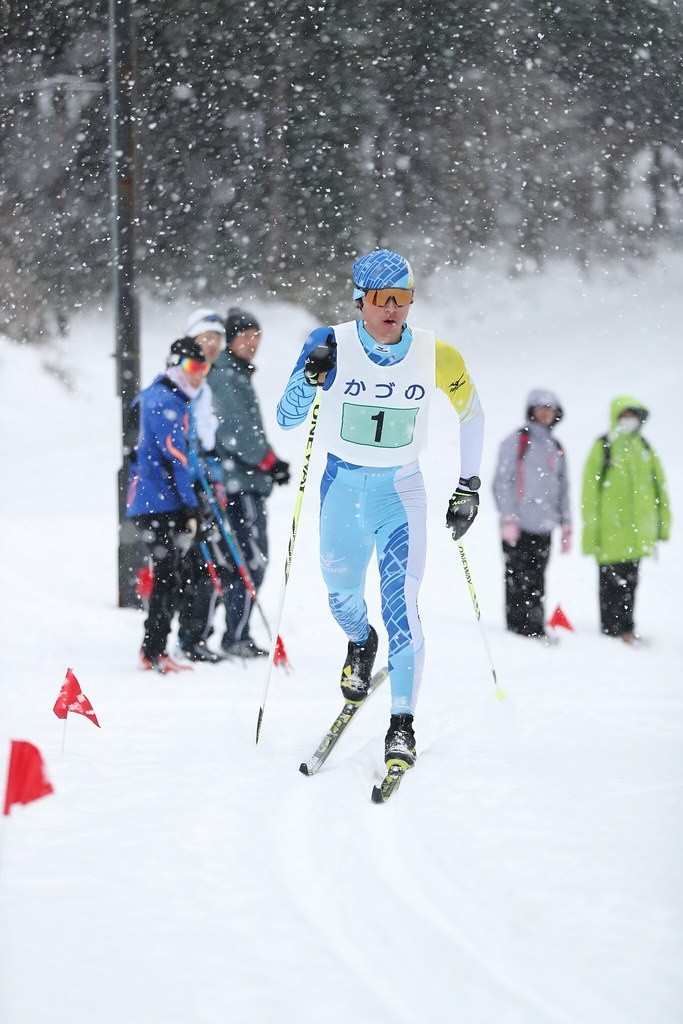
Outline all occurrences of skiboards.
[298,665,405,805]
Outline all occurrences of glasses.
[364,285,413,308]
[203,314,225,325]
[180,355,211,378]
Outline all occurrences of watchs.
[459,476,481,491]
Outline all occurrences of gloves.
[502,523,520,547]
[268,460,289,485]
[446,487,479,541]
[183,506,207,541]
[562,527,571,551]
[304,334,337,375]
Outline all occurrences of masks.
[621,416,640,434]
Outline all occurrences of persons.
[126,306,291,674]
[277,248,485,774]
[581,396,671,640]
[493,388,572,639]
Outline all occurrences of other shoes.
[384,713,416,771]
[186,644,222,664]
[225,639,270,659]
[339,625,378,701]
[141,645,193,675]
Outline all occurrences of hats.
[352,249,413,304]
[187,307,225,340]
[170,337,206,368]
[225,307,259,342]
